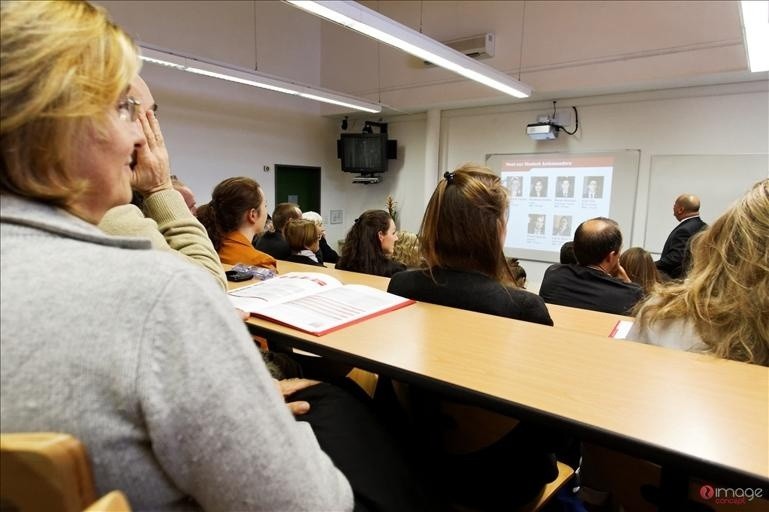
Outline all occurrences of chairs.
[1,432,131,512]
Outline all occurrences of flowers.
[383,195,397,223]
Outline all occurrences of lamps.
[282,0,533,100]
[127,0,384,114]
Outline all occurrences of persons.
[1,1,455,512]
[560,241,579,269]
[385,162,554,327]
[557,177,574,197]
[628,179,769,366]
[617,246,660,293]
[584,176,602,198]
[505,176,522,197]
[653,192,711,279]
[531,176,548,197]
[128,70,252,323]
[528,215,545,233]
[249,200,425,278]
[538,216,643,317]
[194,176,276,275]
[554,215,571,235]
[169,182,199,219]
[498,257,527,290]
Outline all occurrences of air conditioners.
[411,31,494,68]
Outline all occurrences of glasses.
[113,96,140,122]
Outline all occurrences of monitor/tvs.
[340,133,386,172]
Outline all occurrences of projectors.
[527,124,558,141]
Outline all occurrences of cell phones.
[226,270,251,280]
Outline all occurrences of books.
[226,271,415,337]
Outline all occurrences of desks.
[217,258,769,512]
